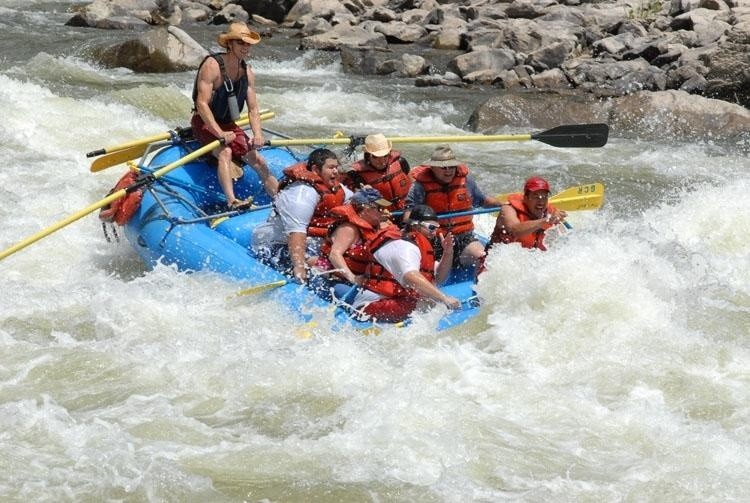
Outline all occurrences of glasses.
[410,217,440,230]
[372,154,389,158]
[370,205,384,213]
[527,193,548,201]
[440,166,456,170]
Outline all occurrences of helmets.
[409,205,439,222]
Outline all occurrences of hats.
[420,143,466,167]
[524,177,552,195]
[361,133,393,158]
[349,187,393,207]
[216,22,262,48]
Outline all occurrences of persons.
[309,186,399,312]
[470,175,569,309]
[191,19,282,212]
[402,145,510,287]
[348,204,465,325]
[342,132,419,229]
[251,148,350,287]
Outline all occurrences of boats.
[124,137,479,337]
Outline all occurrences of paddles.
[269,123,612,151]
[241,274,317,296]
[301,284,357,338]
[363,299,469,340]
[394,191,521,228]
[436,183,609,226]
[90,111,276,173]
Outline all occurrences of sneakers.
[226,196,254,211]
